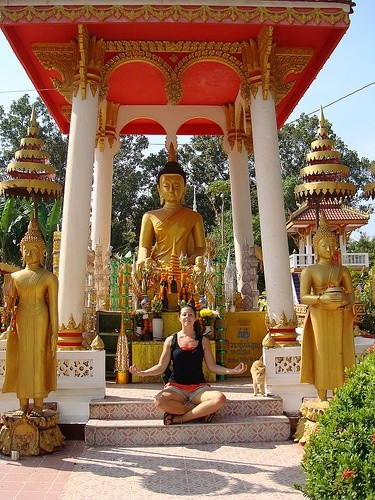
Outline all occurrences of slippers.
[163,413,183,425]
[201,413,215,423]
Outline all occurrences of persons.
[300,209,359,404]
[128,302,248,425]
[135,146,208,311]
[0,217,58,418]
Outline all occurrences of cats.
[249,355,266,398]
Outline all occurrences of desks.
[132,340,216,384]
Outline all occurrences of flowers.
[200,309,213,323]
[150,295,163,314]
[134,309,147,319]
[178,298,193,309]
